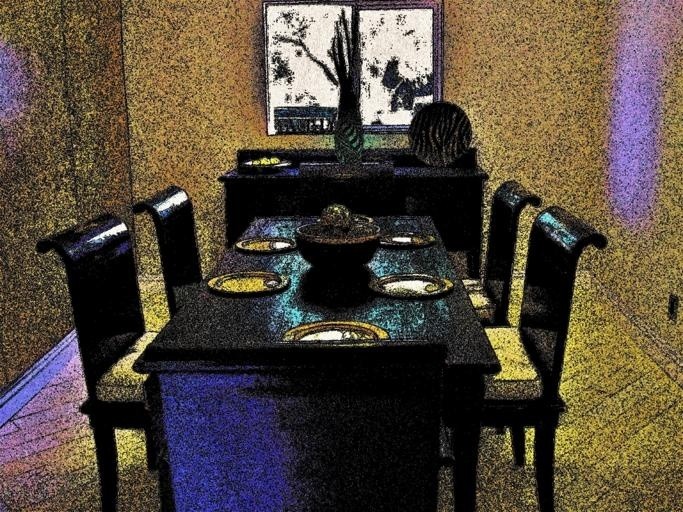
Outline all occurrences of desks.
[219,149,487,281]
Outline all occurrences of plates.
[239,159,292,175]
[379,232,435,248]
[316,213,374,223]
[369,272,454,299]
[234,236,296,254]
[281,320,391,342]
[408,100,473,168]
[208,271,291,296]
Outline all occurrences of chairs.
[130,182,202,325]
[142,344,446,509]
[462,181,544,433]
[36,209,162,510]
[440,204,610,510]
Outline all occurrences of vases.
[333,87,367,163]
